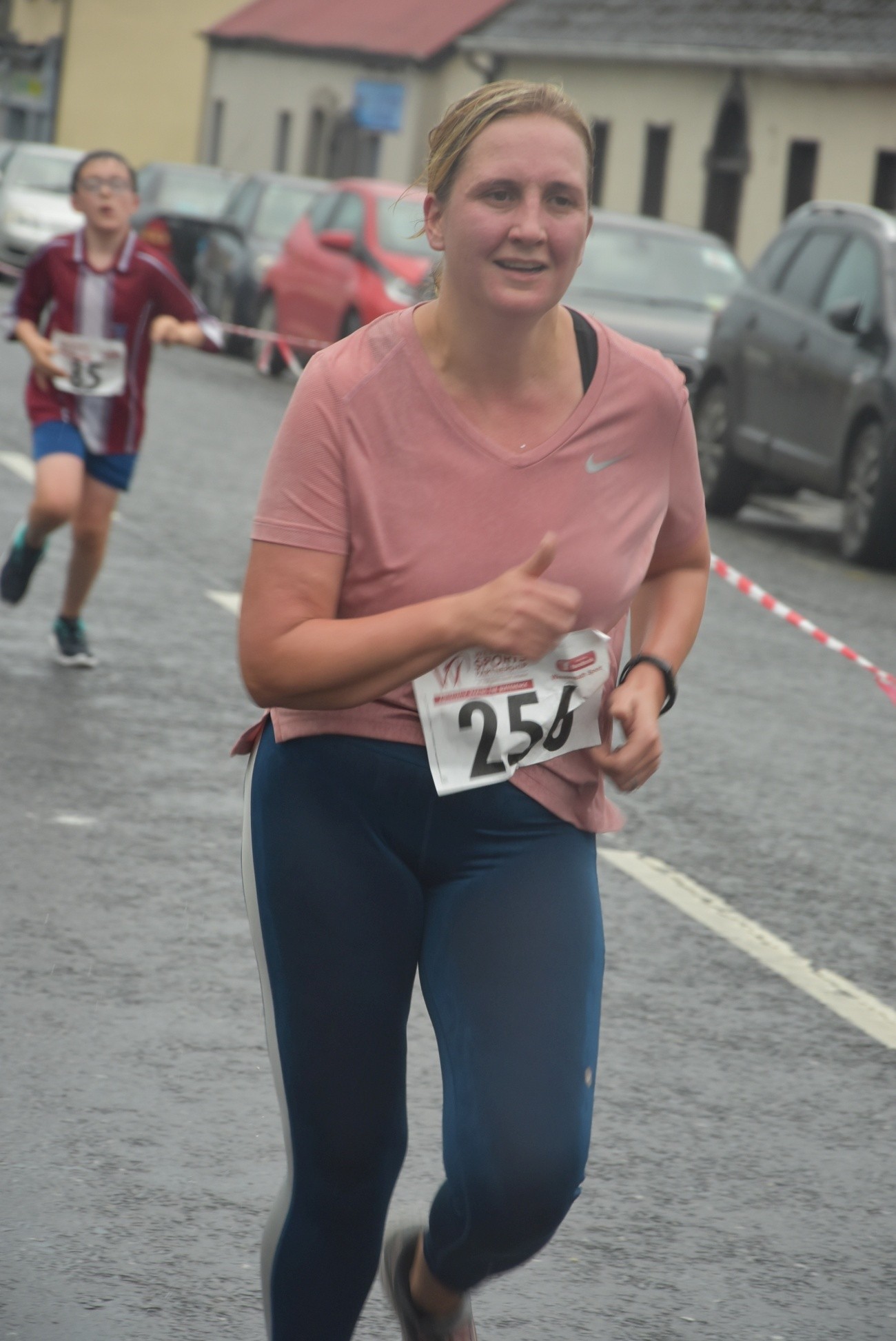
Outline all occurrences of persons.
[3,146,217,670]
[244,77,708,1341]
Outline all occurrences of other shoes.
[383,1227,476,1341]
[48,614,99,670]
[0,519,46,603]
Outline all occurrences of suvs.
[676,197,894,565]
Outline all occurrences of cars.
[0,141,114,284]
[550,211,754,392]
[187,172,324,358]
[125,158,229,285]
[250,176,444,385]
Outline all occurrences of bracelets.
[619,653,678,718]
